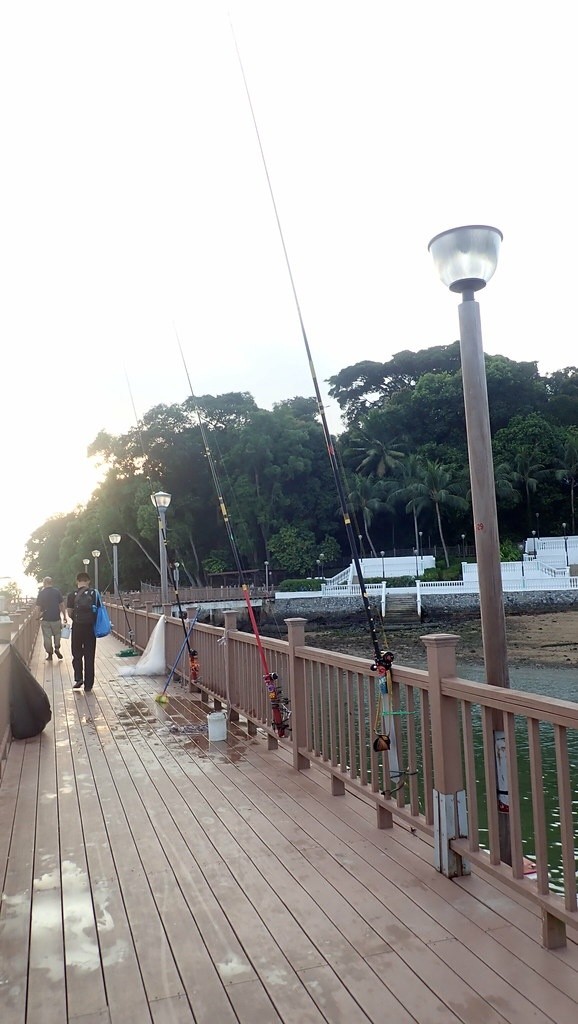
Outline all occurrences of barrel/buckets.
[206,712,227,742]
[60,623,70,639]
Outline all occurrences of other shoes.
[46,655,53,661]
[84,687,91,691]
[55,648,63,659]
[73,678,83,688]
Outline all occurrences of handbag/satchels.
[91,592,112,638]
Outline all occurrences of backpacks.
[74,588,96,625]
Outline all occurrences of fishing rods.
[98,526,134,647]
[239,61,397,677]
[130,390,197,684]
[179,341,288,733]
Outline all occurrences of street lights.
[149,490,172,616]
[82,559,90,575]
[561,522,569,566]
[381,551,386,581]
[264,560,268,591]
[173,562,180,591]
[358,535,363,563]
[535,513,540,537]
[108,533,121,600]
[532,530,537,559]
[424,222,512,866]
[413,549,420,580]
[461,534,467,562]
[315,553,324,579]
[419,531,423,560]
[91,549,100,591]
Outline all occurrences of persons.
[66,573,101,693]
[36,575,68,662]
